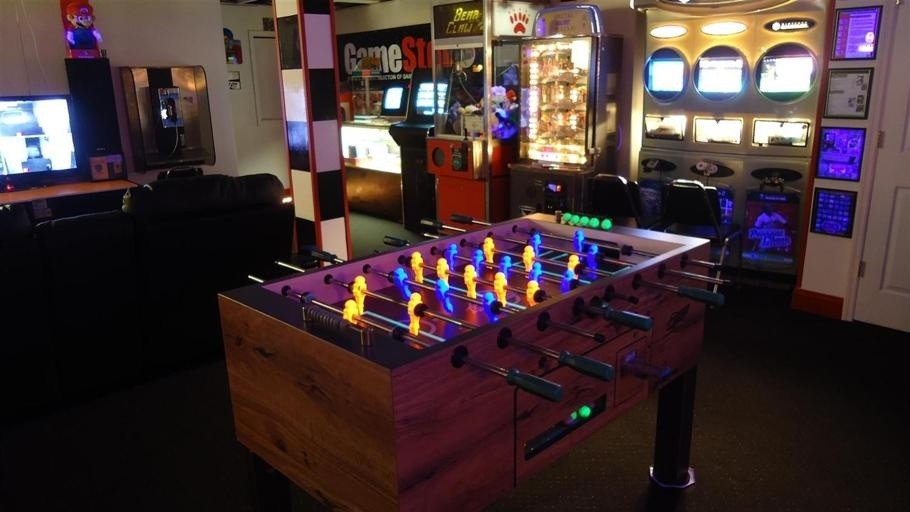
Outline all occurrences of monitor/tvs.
[756,52,815,96]
[694,53,746,96]
[411,80,449,118]
[645,54,687,98]
[381,84,404,112]
[0,93,87,193]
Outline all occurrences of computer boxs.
[63,56,129,184]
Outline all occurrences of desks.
[0,179,137,212]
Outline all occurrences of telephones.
[150,85,184,130]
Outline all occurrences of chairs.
[660,177,743,298]
[591,174,658,231]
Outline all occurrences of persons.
[63,1,103,58]
[223,28,243,64]
